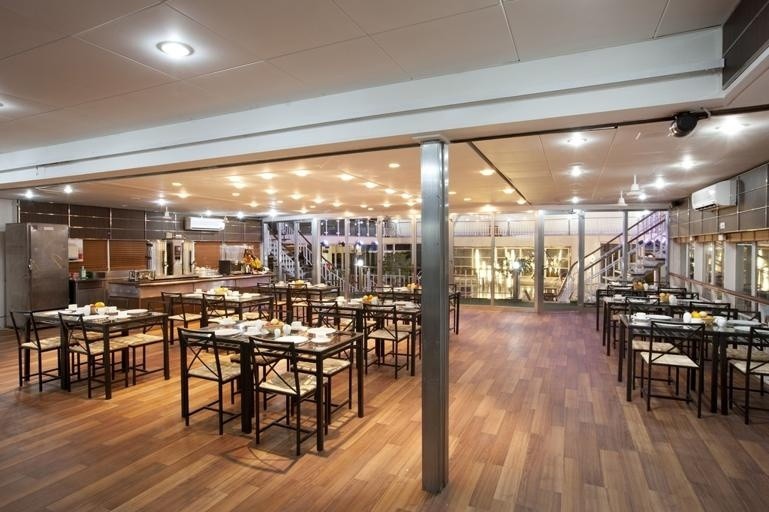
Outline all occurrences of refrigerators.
[4,222,70,331]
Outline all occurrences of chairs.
[597,281,769,425]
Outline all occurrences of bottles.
[245,264,250,273]
[79,266,87,279]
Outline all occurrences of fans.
[617,175,658,198]
[603,188,649,209]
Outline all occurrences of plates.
[383,287,422,294]
[347,302,416,309]
[56,307,148,320]
[210,320,336,344]
[646,314,769,334]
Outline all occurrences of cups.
[196,288,249,297]
[643,282,649,291]
[336,296,411,306]
[613,294,722,306]
[633,311,728,328]
[278,281,324,288]
[68,304,127,318]
[221,318,326,340]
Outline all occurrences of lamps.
[670,110,709,137]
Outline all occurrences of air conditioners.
[185,217,225,232]
[691,178,740,213]
